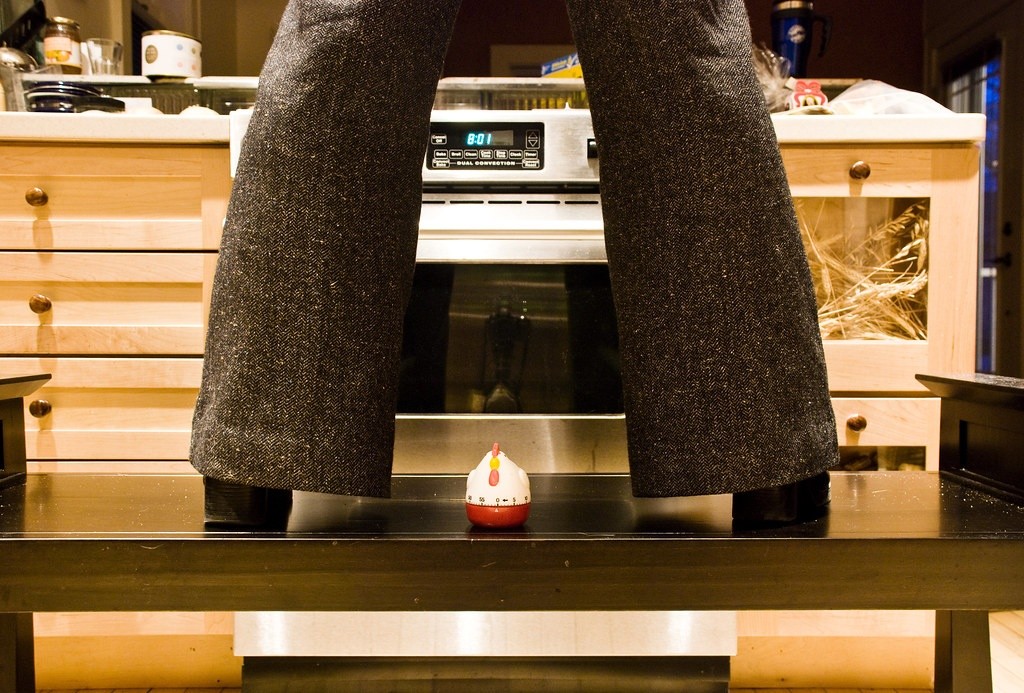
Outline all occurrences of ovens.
[231,110,633,476]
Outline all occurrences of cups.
[87,38,124,74]
[773,0,829,79]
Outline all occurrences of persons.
[190,0,841,528]
[398,264,624,413]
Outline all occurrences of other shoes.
[202,472,289,525]
[734,472,830,522]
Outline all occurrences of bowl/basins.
[141,29,203,78]
